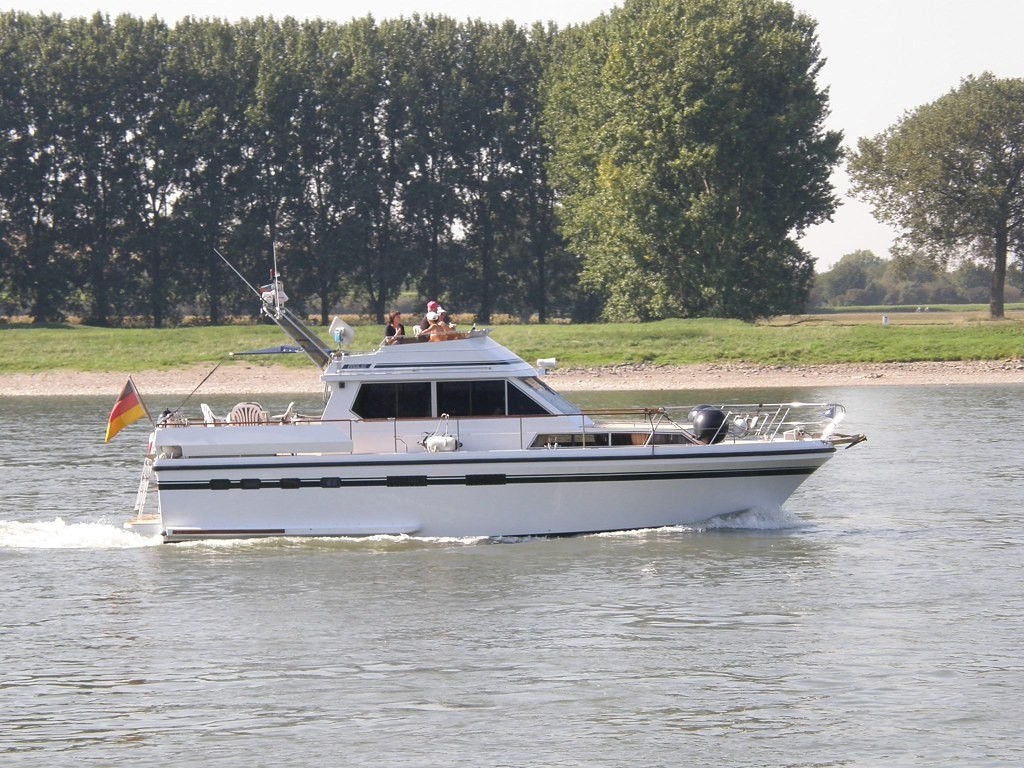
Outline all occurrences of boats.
[123,234,867,544]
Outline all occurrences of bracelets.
[393,337,396,340]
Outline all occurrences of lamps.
[536,357,557,375]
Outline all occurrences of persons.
[383,310,405,345]
[416,301,464,342]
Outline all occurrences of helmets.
[694,407,729,443]
[688,404,712,422]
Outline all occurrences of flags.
[105,379,146,444]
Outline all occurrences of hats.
[437,307,447,314]
[428,301,438,311]
[426,311,439,321]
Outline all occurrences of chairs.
[272,401,296,426]
[227,402,269,427]
[201,403,225,427]
